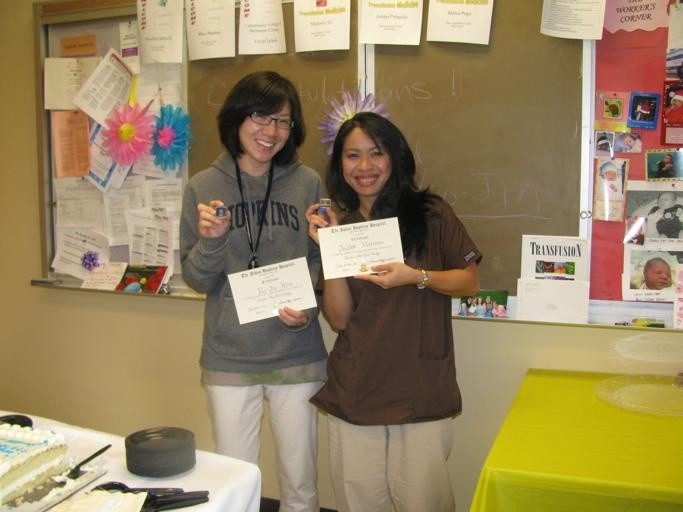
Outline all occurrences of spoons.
[68,444,113,480]
[89,481,212,511]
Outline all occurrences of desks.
[0,410,262,512]
[470,368,683,512]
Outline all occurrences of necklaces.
[233,158,273,269]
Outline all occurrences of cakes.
[0,423,73,508]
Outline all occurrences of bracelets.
[417,267,430,290]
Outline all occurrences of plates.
[125,425,198,480]
[596,371,682,417]
[1,415,33,429]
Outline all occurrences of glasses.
[246,111,295,129]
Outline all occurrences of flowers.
[317,87,393,155]
[101,103,194,172]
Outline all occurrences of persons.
[302,111,483,511]
[599,162,620,196]
[636,256,674,292]
[605,102,619,117]
[663,90,683,126]
[594,136,609,154]
[177,68,333,510]
[630,98,656,124]
[457,294,505,318]
[653,152,673,180]
[616,133,642,153]
[643,190,683,245]
